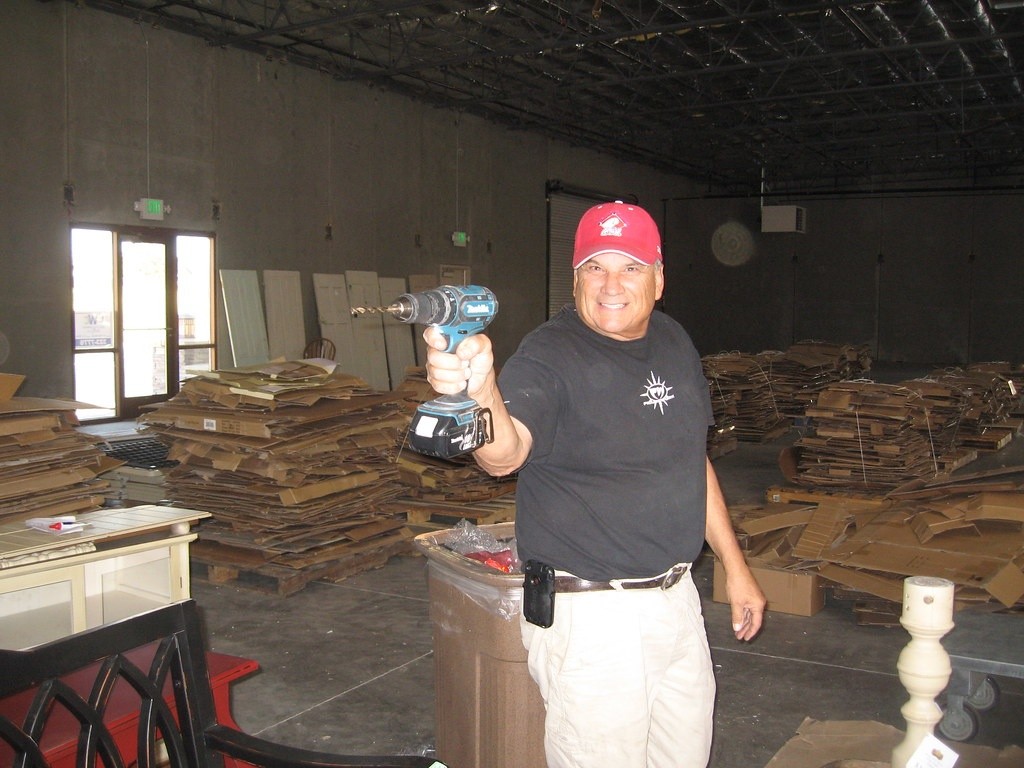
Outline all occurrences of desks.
[0,640,260,768]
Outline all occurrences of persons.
[423,200,766,768]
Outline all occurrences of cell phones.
[524,560,556,629]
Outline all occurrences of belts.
[555,567,687,593]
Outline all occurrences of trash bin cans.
[410,521,550,768]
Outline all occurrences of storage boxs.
[713,559,827,616]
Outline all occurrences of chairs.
[0,601,449,768]
[303,338,336,361]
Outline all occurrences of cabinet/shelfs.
[0,505,213,652]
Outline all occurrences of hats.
[571,200,663,270]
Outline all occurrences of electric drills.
[350,284,498,459]
